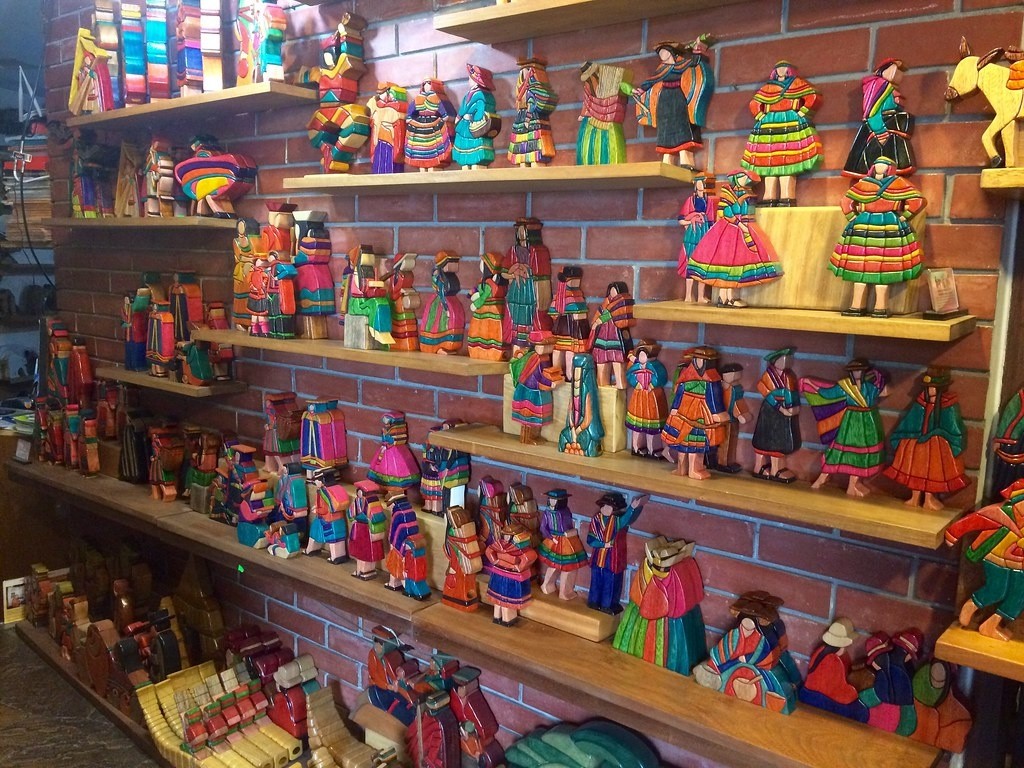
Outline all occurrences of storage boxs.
[712,206,927,318]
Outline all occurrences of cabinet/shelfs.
[0,0,1024,768]
